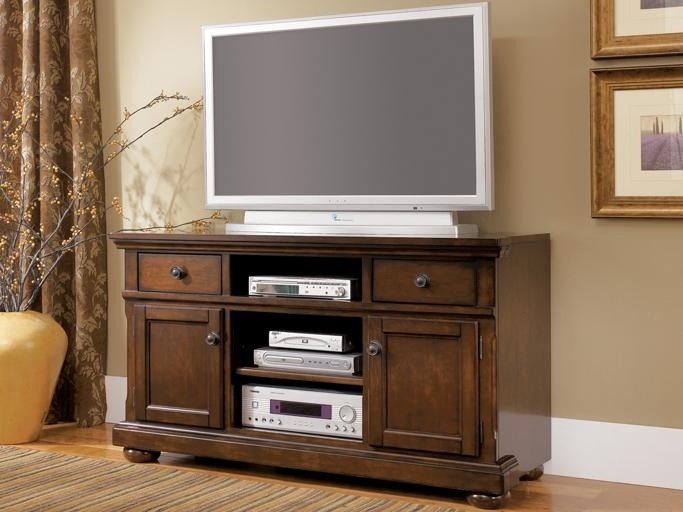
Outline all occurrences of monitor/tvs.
[203,2,495,237]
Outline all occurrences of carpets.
[0,439,460,512]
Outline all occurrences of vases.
[1,310,71,445]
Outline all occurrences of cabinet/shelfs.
[107,233,552,504]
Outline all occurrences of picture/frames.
[579,62,682,220]
[585,0,682,60]
